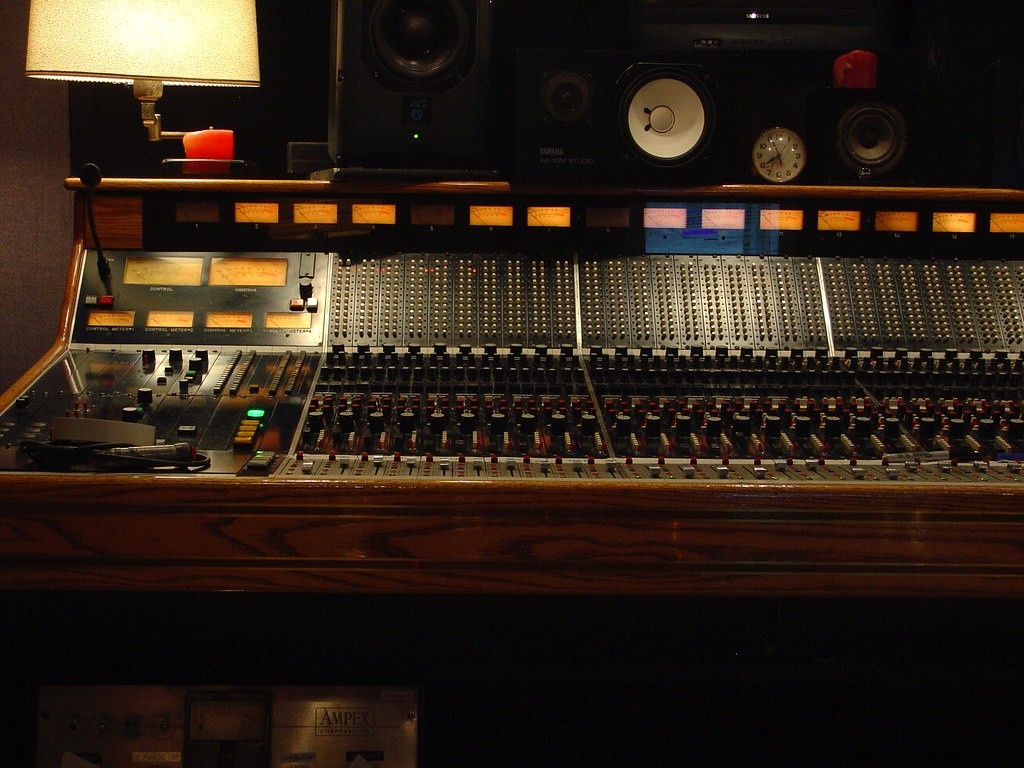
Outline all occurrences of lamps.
[26,0,261,139]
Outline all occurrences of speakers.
[511,47,921,187]
[327,0,505,170]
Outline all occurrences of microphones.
[79,164,112,281]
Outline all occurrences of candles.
[183,125,234,177]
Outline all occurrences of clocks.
[753,127,810,183]
